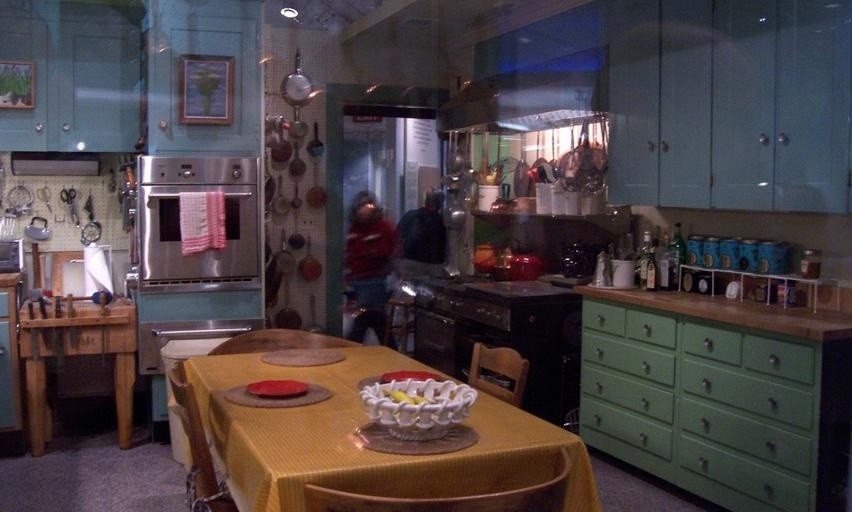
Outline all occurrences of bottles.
[669,221,686,287]
[592,251,612,286]
[646,247,658,292]
[492,247,512,280]
[798,248,821,281]
[640,230,653,285]
[555,241,590,278]
[659,251,673,290]
[472,242,498,273]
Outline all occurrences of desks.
[16,295,138,458]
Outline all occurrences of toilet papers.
[83,242,114,303]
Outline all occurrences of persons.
[393,186,449,281]
[336,191,400,352]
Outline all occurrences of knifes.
[54,295,64,370]
[66,292,79,351]
[36,295,52,351]
[27,303,39,361]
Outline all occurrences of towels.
[178,190,228,256]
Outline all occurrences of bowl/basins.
[442,204,465,232]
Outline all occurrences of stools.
[386,298,418,353]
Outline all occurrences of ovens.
[137,154,267,377]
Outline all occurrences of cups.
[478,183,500,212]
[535,183,602,215]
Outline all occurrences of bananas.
[384,388,415,405]
[414,395,424,405]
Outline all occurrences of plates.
[244,380,309,398]
[381,370,442,383]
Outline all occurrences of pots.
[265,47,327,336]
[508,253,542,279]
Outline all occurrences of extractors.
[437,46,613,139]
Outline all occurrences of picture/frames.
[0,60,36,110]
[178,54,234,127]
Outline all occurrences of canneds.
[800,248,823,279]
[777,279,797,305]
[685,234,792,275]
[756,283,773,302]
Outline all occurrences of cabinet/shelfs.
[676,303,851,511]
[607,0,713,212]
[712,0,852,212]
[572,285,679,487]
[0,273,30,454]
[141,1,267,159]
[1,11,144,153]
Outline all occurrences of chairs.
[168,360,237,510]
[304,446,574,510]
[469,343,531,408]
[208,328,362,356]
